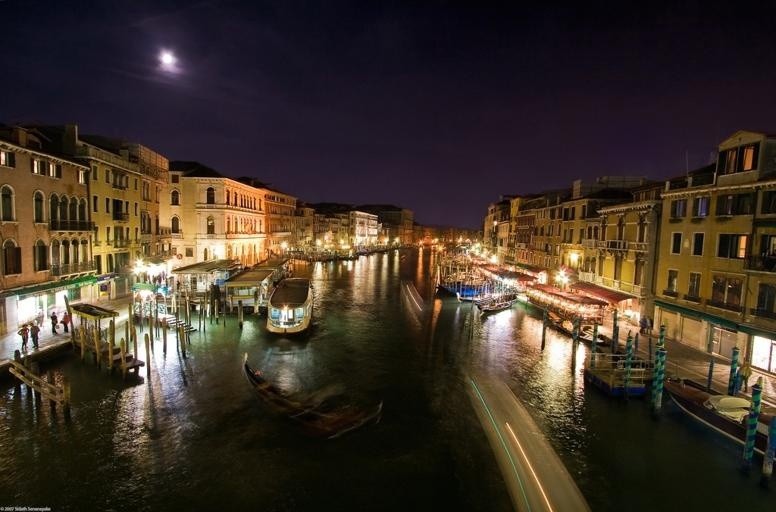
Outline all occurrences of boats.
[584,352,647,397]
[439,249,518,311]
[664,378,776,463]
[265,277,314,337]
[242,352,383,437]
[547,312,605,345]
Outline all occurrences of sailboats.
[295,244,423,262]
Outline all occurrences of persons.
[639,314,647,334]
[50,311,59,336]
[646,315,654,336]
[737,358,752,394]
[17,324,29,352]
[61,312,71,333]
[29,322,40,349]
